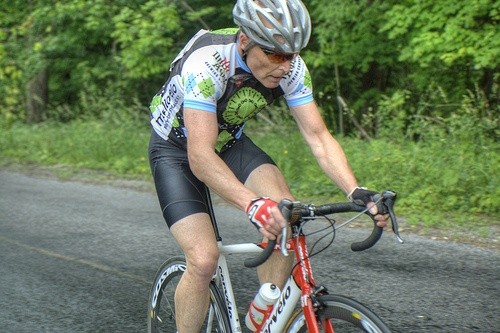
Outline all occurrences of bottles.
[245,282,282,333]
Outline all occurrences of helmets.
[232,0,312,54]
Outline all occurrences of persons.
[148,0,390,333]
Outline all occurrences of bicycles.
[146,186,404,333]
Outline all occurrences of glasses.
[260,48,300,64]
